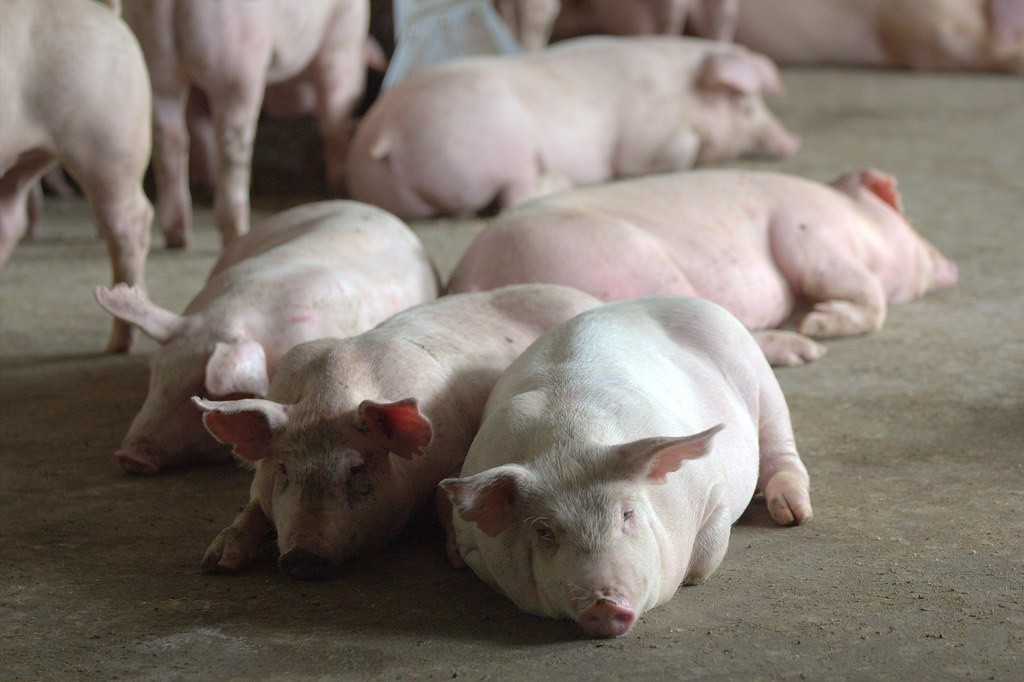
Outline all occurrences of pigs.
[0,0,1024,637]
